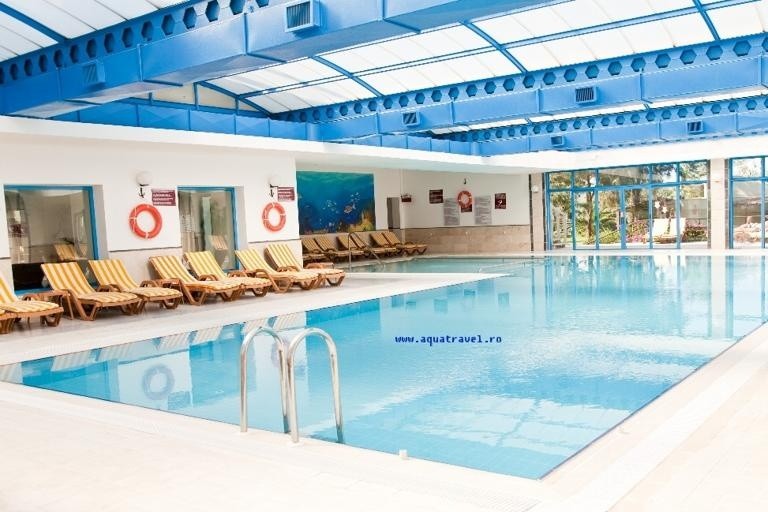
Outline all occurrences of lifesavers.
[457,191,472,208]
[262,202,286,233]
[128,204,162,239]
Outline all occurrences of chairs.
[660,218,686,244]
[643,218,669,243]
